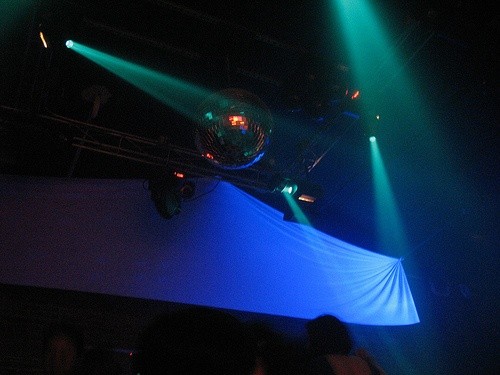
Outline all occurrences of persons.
[36,314,388,375]
